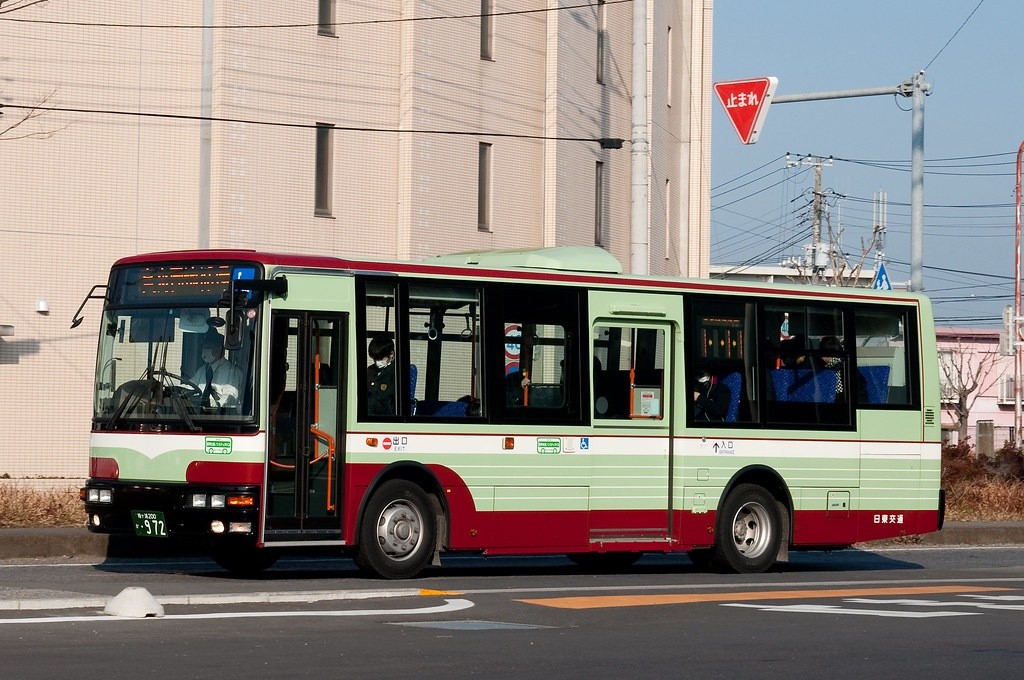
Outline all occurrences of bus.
[69,243,942,578]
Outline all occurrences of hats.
[200,332,224,344]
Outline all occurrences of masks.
[696,376,710,382]
[374,358,392,369]
[202,348,220,364]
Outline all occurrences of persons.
[367,336,397,416]
[819,337,869,403]
[190,333,245,413]
[694,356,731,422]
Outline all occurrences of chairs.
[412,365,892,425]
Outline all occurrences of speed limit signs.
[503,325,523,359]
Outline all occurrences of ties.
[203,366,213,397]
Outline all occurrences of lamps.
[35,298,49,312]
